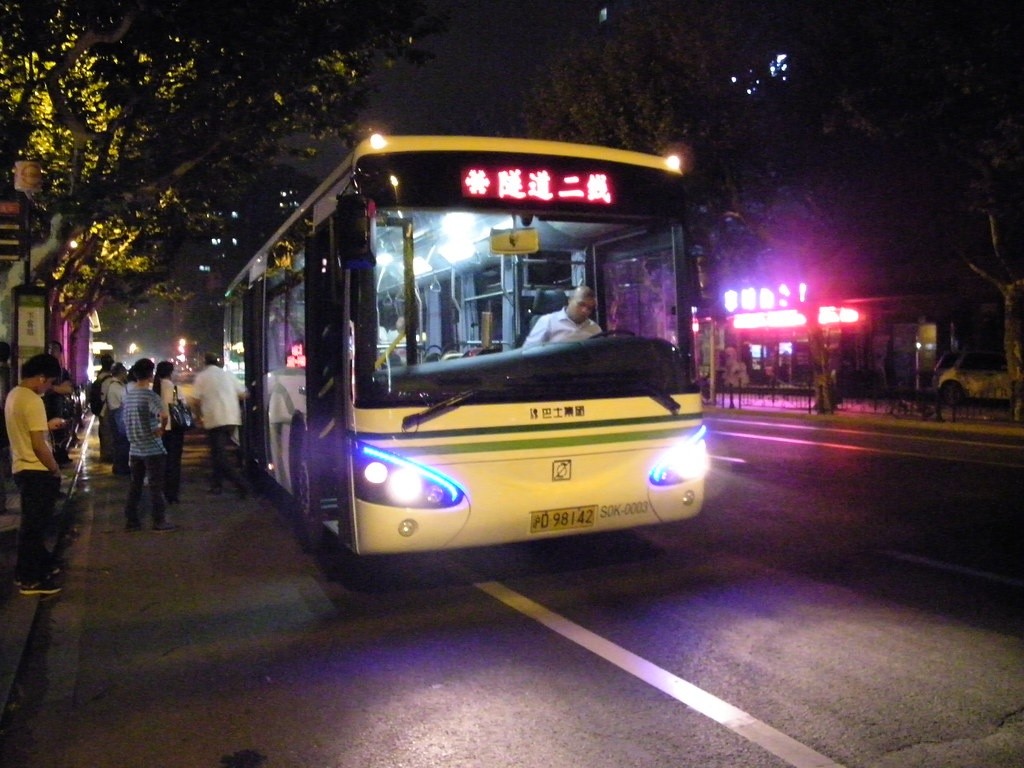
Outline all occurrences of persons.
[106,362,137,478]
[153,361,185,504]
[95,355,113,464]
[523,286,602,346]
[122,359,176,533]
[192,353,250,495]
[4,340,72,594]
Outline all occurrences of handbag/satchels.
[168,385,195,432]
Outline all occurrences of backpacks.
[87,375,114,415]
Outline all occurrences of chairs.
[528,288,569,333]
[374,339,510,369]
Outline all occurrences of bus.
[220,130,713,561]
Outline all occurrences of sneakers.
[19,580,62,594]
[15,573,50,586]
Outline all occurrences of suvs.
[932,347,1014,404]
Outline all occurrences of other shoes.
[112,464,131,474]
[152,521,177,532]
[126,520,146,531]
